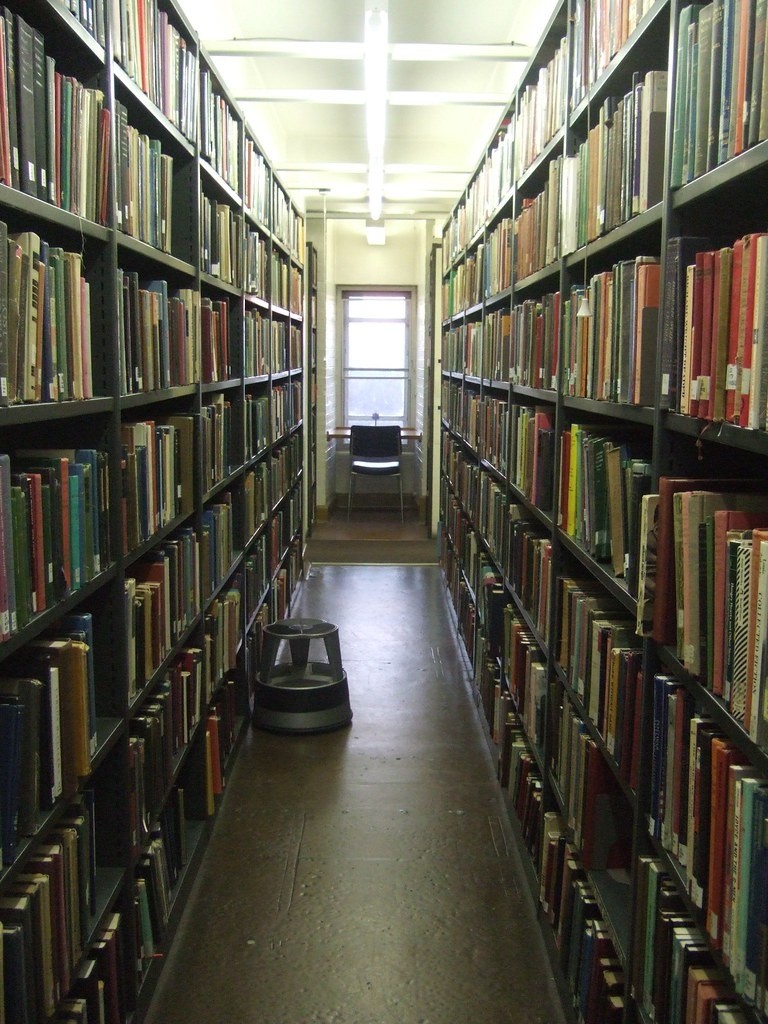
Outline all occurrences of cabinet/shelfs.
[0,1,321,1024]
[429,1,768,1024]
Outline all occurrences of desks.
[325,428,423,508]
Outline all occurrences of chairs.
[344,422,405,523]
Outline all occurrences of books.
[0,0,318,1024]
[438,0,768,1024]
